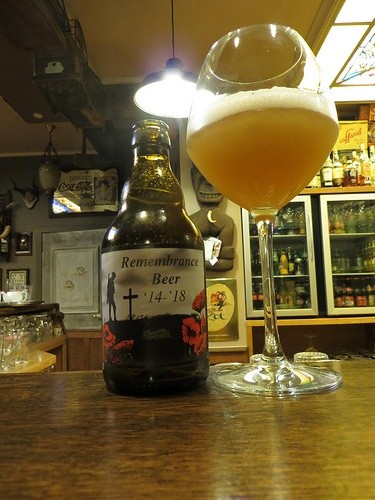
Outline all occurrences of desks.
[0,357,375,500]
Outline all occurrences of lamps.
[133,1,199,118]
[38,124,62,202]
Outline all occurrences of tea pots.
[0,291,27,304]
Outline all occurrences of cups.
[0,312,53,372]
[6,279,32,302]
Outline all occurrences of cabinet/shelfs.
[319,193,375,315]
[242,196,319,316]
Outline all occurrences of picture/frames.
[6,268,30,285]
[14,231,32,257]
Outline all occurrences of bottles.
[252,246,308,275]
[329,202,375,234]
[100,120,210,398]
[249,206,306,235]
[305,144,375,188]
[333,277,375,308]
[331,240,375,273]
[252,278,311,308]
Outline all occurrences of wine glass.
[185,23,344,398]
